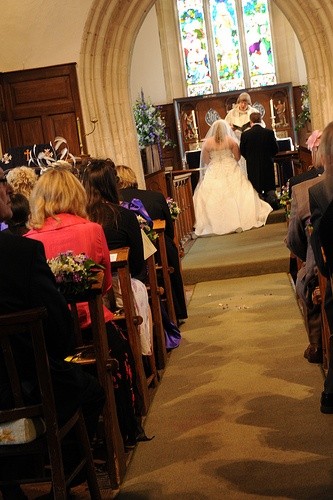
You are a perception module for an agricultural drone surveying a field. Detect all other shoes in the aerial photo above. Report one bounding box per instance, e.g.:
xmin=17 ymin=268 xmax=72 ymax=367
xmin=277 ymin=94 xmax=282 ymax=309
xmin=304 ymin=343 xmax=323 ymax=363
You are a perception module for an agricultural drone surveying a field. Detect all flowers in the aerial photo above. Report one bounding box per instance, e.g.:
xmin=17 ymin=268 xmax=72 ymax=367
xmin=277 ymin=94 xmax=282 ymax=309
xmin=136 ymin=214 xmax=160 ymax=242
xmin=276 ymin=180 xmax=293 ymax=219
xmin=294 ymin=84 xmax=311 ymax=131
xmin=46 ymin=249 xmax=106 ymax=296
xmin=305 ymin=130 xmax=323 ymax=151
xmin=166 ymin=196 xmax=181 ymax=220
xmin=305 ymin=217 xmax=314 ymax=237
xmin=134 ymin=91 xmax=178 ymax=150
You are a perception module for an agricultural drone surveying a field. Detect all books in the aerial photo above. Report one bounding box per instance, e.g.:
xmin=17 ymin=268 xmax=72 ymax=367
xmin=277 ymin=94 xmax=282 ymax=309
xmin=232 ymin=121 xmax=251 ymax=133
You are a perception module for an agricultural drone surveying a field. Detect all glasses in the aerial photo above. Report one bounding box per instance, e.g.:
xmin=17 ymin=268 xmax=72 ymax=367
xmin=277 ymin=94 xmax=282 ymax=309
xmin=0 ymin=176 xmax=7 ymax=184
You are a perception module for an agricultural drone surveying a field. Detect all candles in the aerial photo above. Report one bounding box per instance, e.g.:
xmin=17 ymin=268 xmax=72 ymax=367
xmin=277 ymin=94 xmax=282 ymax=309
xmin=191 ymin=110 xmax=196 ymax=128
xmin=269 ymin=99 xmax=274 ymax=117
xmin=76 ymin=116 xmax=83 ymax=146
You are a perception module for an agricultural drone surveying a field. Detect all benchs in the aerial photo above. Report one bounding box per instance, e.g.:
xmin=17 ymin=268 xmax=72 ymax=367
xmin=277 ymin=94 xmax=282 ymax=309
xmin=0 ymin=221 xmax=188 ymax=500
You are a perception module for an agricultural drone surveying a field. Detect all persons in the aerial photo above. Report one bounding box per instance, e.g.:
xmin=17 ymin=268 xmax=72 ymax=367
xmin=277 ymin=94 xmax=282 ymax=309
xmin=0 ymin=159 xmax=185 ymax=463
xmin=224 ymin=91 xmax=267 ymax=140
xmin=239 ymin=111 xmax=283 ymax=211
xmin=286 ymin=119 xmax=333 ymax=364
xmin=192 ymin=119 xmax=274 ymax=237
